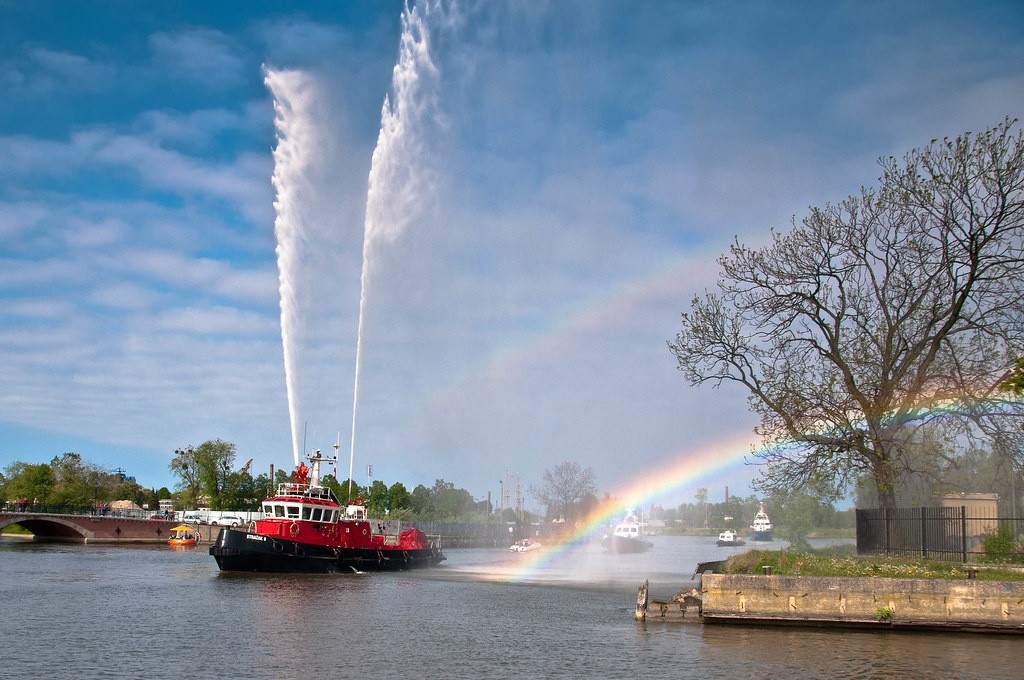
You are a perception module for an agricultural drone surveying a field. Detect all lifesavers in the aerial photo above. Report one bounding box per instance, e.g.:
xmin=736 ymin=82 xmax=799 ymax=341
xmin=294 ymin=547 xmax=306 ymax=559
xmin=382 ymin=557 xmax=392 ymax=569
xmin=290 ymin=523 xmax=299 ymax=535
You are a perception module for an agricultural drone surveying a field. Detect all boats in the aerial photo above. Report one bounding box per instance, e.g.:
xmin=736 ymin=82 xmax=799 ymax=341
xmin=749 ymin=500 xmax=774 ymax=542
xmin=167 ymin=522 xmax=202 ymax=548
xmin=602 ymin=506 xmax=657 ymax=555
xmin=716 ymin=525 xmax=747 ymax=547
xmin=508 ymin=538 xmax=543 ymax=554
xmin=209 ymin=421 xmax=448 ymax=576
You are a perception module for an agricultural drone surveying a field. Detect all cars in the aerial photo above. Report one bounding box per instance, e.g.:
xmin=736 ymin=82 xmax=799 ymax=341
xmin=179 ymin=514 xmax=207 ymax=525
xmin=207 ymin=514 xmax=245 ymax=529
xmin=147 ymin=514 xmax=172 ymax=522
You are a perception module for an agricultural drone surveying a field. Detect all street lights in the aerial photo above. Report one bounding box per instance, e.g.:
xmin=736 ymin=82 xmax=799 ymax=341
xmin=94 ymin=478 xmax=99 ymax=515
xmin=499 ymin=481 xmax=503 ymax=526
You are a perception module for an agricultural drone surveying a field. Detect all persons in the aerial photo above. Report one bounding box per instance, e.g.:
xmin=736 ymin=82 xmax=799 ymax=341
xmin=607 ymin=525 xmax=613 ymax=535
xmin=34 ymin=498 xmax=39 ymax=512
xmin=293 ymin=462 xmax=309 ymax=491
xmin=377 ymin=522 xmax=386 ymax=535
xmin=96 ymin=501 xmax=109 ymax=516
xmin=515 ymin=540 xmax=529 ymax=546
xmin=165 ymin=508 xmax=175 ymax=521
xmin=169 ymin=534 xmax=192 ymax=539
xmin=356 ymin=496 xmax=363 ymax=506
xmin=18 ymin=498 xmax=28 ymax=513
